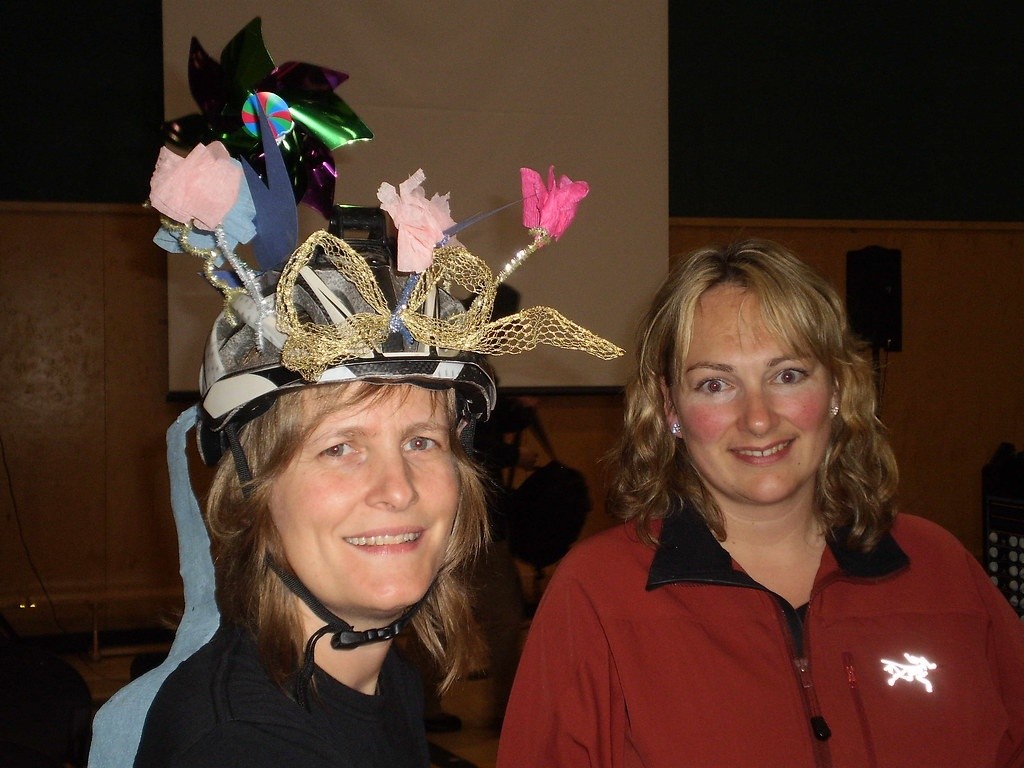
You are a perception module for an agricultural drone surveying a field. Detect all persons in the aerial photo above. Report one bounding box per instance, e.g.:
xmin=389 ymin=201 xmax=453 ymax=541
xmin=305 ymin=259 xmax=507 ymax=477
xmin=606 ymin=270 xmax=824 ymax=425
xmin=69 ymin=239 xmax=492 ymax=768
xmin=492 ymin=239 xmax=1024 ymax=768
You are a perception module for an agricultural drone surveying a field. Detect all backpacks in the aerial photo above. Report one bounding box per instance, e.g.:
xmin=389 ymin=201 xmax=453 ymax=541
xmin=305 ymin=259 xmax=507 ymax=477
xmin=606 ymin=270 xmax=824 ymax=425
xmin=501 ymin=407 xmax=592 ymax=570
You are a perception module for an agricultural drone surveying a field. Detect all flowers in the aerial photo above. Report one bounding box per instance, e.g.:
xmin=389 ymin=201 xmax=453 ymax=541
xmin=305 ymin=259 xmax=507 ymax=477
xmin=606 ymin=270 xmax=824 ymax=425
xmin=149 ymin=140 xmax=277 ymax=355
xmin=376 ymin=167 xmax=466 ymax=274
xmin=153 ymin=157 xmax=265 ymax=303
xmin=455 ymin=163 xmax=590 ymax=322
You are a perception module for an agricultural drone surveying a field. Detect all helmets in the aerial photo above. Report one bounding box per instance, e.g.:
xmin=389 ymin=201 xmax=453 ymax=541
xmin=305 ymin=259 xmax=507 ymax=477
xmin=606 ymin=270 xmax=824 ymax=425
xmin=194 ymin=264 xmax=500 ymax=469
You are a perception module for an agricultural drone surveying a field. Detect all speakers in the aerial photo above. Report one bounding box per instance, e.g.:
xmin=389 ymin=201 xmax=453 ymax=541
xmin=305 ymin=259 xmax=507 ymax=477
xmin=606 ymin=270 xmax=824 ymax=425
xmin=843 ymin=245 xmax=904 ymax=352
